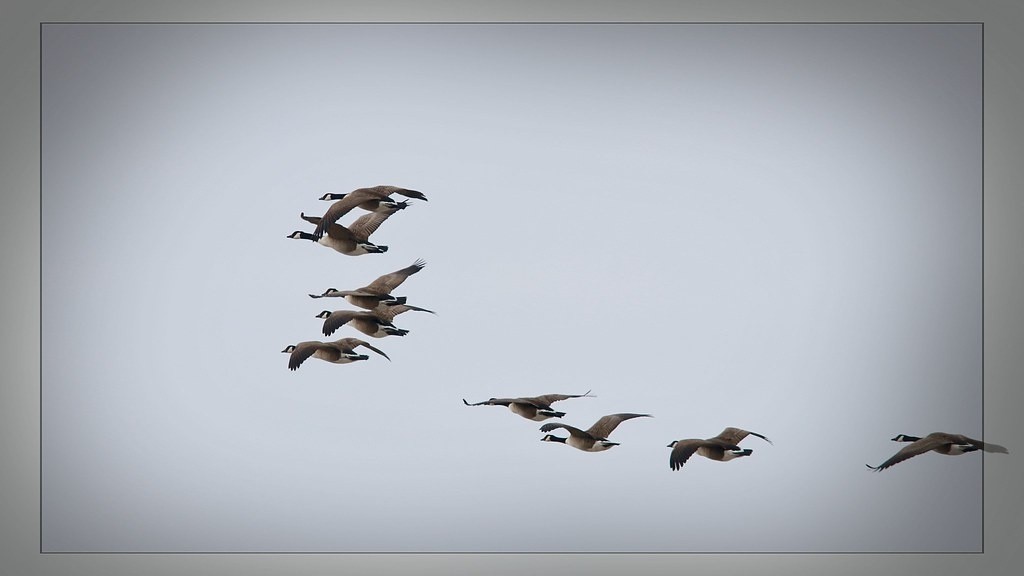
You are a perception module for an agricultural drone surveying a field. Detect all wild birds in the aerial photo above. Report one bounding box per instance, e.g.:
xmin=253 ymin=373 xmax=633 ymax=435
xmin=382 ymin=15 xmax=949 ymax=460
xmin=667 ymin=427 xmax=774 ymax=471
xmin=462 ymin=389 xmax=597 ymax=422
xmin=866 ymin=433 xmax=1009 ymax=472
xmin=287 ymin=186 xmax=428 ymax=256
xmin=539 ymin=413 xmax=655 ymax=453
xmin=281 ymin=258 xmax=437 ymax=372
